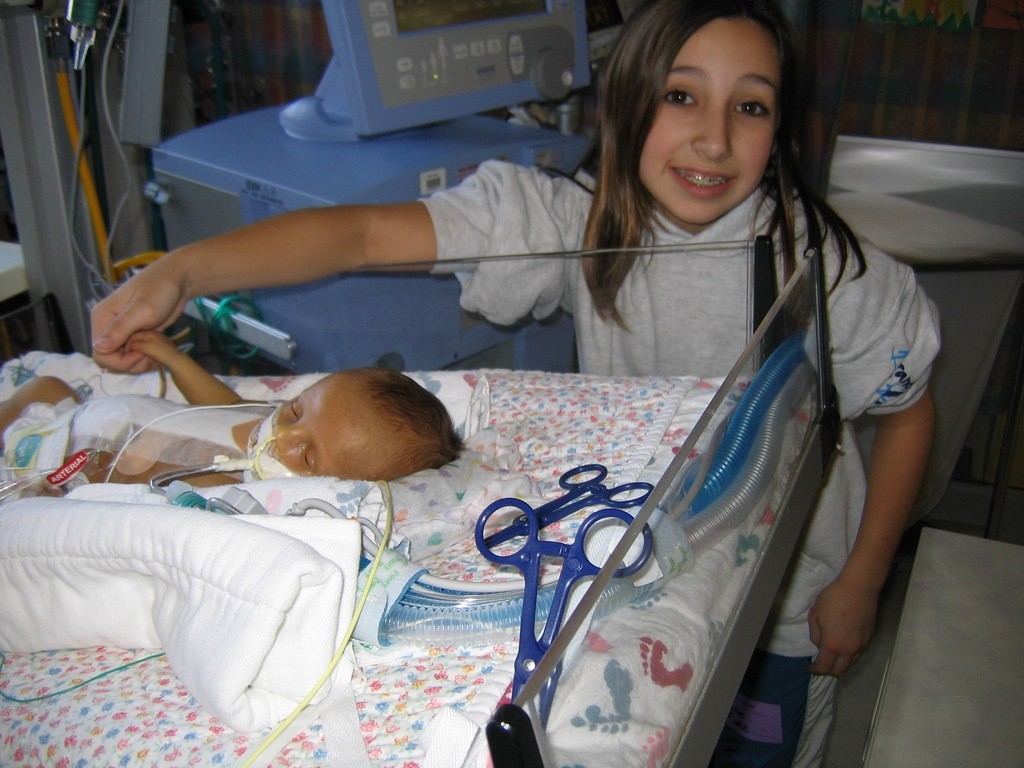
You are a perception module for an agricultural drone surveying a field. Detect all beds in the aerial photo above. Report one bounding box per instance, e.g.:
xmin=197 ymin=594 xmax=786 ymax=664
xmin=0 ymin=236 xmax=841 ymax=768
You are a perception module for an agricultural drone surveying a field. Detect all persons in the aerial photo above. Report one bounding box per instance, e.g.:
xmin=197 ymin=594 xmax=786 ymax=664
xmin=0 ymin=327 xmax=466 ymax=502
xmin=88 ymin=0 xmax=943 ymax=768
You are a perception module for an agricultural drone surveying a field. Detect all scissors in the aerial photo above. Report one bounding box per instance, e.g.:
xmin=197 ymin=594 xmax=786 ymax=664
xmin=472 ymin=496 xmax=655 ymax=731
xmin=478 ymin=463 xmax=658 ymax=550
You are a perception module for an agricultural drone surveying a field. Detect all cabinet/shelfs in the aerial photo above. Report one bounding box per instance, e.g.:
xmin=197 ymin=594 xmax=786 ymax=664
xmin=148 ymin=95 xmax=593 ymax=377
